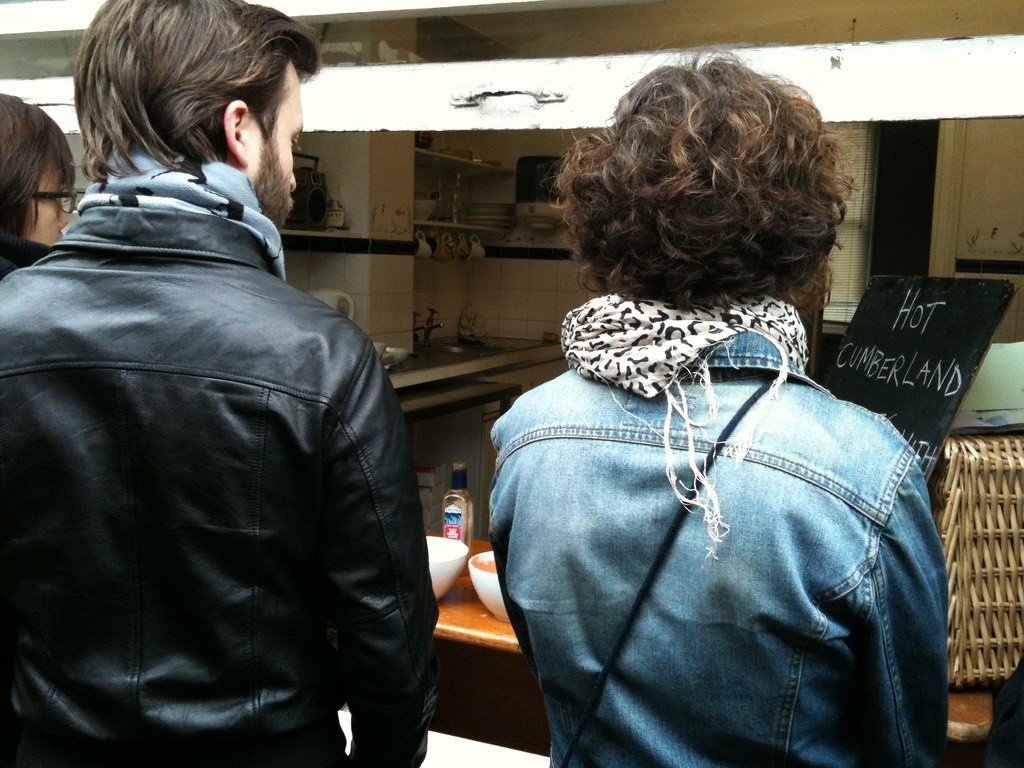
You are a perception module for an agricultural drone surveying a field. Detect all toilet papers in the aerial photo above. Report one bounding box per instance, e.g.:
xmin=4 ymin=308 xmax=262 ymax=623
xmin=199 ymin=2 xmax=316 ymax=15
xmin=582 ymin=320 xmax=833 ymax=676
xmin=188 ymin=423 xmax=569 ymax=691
xmin=304 ymin=285 xmax=361 ymax=327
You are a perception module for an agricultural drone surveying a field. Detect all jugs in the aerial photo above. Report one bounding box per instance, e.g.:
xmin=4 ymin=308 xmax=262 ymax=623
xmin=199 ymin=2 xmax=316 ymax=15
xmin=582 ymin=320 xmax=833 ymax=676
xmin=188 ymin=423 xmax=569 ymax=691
xmin=306 ymin=288 xmax=355 ymax=322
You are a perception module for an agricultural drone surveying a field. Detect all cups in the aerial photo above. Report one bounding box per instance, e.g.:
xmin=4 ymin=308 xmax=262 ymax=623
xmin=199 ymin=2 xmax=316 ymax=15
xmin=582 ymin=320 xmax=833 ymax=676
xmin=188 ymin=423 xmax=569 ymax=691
xmin=416 ymin=131 xmax=472 ymax=151
xmin=414 ymin=230 xmax=487 ymax=266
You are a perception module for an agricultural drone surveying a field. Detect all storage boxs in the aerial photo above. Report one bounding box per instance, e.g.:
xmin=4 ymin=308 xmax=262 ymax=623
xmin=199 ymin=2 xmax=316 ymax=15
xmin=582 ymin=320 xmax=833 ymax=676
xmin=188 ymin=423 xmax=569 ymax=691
xmin=926 ymin=432 xmax=1024 ymax=689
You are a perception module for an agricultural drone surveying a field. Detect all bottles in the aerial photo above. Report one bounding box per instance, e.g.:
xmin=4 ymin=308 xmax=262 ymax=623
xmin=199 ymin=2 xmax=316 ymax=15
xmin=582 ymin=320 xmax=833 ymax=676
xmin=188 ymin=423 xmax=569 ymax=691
xmin=447 ymin=168 xmax=466 ymax=225
xmin=441 ymin=462 xmax=473 ymax=576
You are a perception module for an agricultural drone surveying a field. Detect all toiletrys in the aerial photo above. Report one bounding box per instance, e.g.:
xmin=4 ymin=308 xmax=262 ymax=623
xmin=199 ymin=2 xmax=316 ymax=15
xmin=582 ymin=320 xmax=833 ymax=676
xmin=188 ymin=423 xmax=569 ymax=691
xmin=439 ymin=459 xmax=477 ymax=580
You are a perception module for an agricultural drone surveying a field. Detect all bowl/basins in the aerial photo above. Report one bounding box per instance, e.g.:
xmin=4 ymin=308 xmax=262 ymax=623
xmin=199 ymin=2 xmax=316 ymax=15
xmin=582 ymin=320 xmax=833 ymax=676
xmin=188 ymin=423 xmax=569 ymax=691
xmin=414 ymin=197 xmax=436 ymax=222
xmin=467 ymin=549 xmax=511 ymax=622
xmin=425 ymin=535 xmax=470 ymax=601
xmin=440 ymin=148 xmax=473 ymax=160
xmin=372 ymin=341 xmax=409 ymax=372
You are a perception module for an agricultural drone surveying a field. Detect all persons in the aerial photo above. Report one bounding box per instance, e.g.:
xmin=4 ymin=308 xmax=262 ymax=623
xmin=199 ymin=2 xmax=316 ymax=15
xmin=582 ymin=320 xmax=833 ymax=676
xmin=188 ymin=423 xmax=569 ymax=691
xmin=0 ymin=90 xmax=76 ymax=288
xmin=483 ymin=45 xmax=955 ymax=766
xmin=1 ymin=0 xmax=439 ymax=766
xmin=977 ymin=654 xmax=1024 ymax=768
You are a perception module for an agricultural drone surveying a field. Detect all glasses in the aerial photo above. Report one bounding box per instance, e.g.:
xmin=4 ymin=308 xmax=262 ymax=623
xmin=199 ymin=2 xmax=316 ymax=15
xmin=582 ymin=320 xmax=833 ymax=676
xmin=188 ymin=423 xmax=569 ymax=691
xmin=33 ymin=187 xmax=77 ymax=214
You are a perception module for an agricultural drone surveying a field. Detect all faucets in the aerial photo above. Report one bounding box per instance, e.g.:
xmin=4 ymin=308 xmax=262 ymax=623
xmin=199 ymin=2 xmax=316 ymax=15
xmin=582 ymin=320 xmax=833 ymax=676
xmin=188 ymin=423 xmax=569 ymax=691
xmin=413 ymin=310 xmax=430 ymax=348
xmin=425 ymin=308 xmax=445 ymax=345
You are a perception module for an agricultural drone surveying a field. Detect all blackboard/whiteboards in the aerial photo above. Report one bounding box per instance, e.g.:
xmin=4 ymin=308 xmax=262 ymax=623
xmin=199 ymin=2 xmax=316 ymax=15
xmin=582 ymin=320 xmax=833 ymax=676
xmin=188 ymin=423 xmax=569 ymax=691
xmin=819 ymin=274 xmax=1020 ymax=485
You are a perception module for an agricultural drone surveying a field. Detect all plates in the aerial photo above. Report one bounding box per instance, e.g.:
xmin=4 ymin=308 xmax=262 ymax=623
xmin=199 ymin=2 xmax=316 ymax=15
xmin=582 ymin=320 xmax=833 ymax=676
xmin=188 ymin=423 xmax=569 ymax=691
xmin=522 ymin=213 xmax=560 ymax=229
xmin=461 ymin=198 xmax=516 ymax=234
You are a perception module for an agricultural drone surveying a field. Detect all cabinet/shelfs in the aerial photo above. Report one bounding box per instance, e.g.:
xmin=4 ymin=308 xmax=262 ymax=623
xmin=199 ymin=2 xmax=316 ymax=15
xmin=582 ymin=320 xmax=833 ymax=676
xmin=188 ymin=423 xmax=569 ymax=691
xmin=412 ymin=17 xmax=529 ymax=244
xmin=927 ymin=119 xmax=1024 ymax=342
